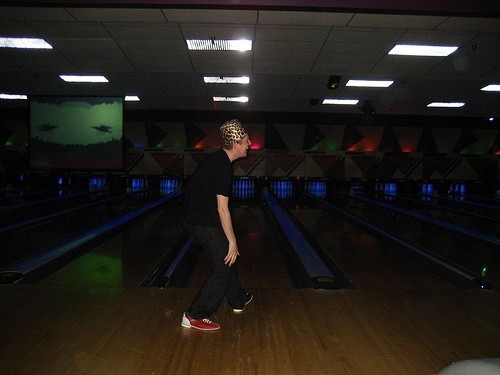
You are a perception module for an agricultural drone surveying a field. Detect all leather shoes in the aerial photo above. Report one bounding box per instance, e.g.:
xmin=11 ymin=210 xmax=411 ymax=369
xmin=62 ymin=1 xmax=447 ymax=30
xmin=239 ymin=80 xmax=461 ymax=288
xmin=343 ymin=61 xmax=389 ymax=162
xmin=181 ymin=311 xmax=220 ymax=330
xmin=233 ymin=293 xmax=253 ymax=312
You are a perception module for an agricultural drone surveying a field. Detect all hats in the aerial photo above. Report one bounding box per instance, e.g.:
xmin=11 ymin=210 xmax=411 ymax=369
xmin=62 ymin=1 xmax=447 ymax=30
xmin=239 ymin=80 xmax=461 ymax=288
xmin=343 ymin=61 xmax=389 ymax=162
xmin=220 ymin=119 xmax=248 ymax=146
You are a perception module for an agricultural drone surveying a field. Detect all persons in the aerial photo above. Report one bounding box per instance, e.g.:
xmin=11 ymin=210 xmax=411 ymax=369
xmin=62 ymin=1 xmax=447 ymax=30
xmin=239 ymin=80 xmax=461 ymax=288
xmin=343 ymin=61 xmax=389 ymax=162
xmin=181 ymin=118 xmax=253 ymax=330
